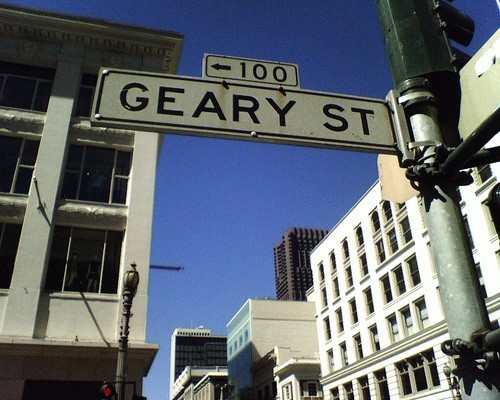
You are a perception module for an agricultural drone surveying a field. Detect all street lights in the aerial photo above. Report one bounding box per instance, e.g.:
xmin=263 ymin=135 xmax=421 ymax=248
xmin=444 ymin=362 xmax=462 ymax=400
xmin=115 ymin=263 xmax=138 ymax=400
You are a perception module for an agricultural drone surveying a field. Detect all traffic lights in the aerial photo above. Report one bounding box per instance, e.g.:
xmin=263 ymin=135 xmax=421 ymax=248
xmin=100 ymin=384 xmax=116 ymax=400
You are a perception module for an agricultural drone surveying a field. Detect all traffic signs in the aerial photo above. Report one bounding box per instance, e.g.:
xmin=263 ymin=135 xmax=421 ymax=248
xmin=89 ymin=53 xmax=400 ymax=155
